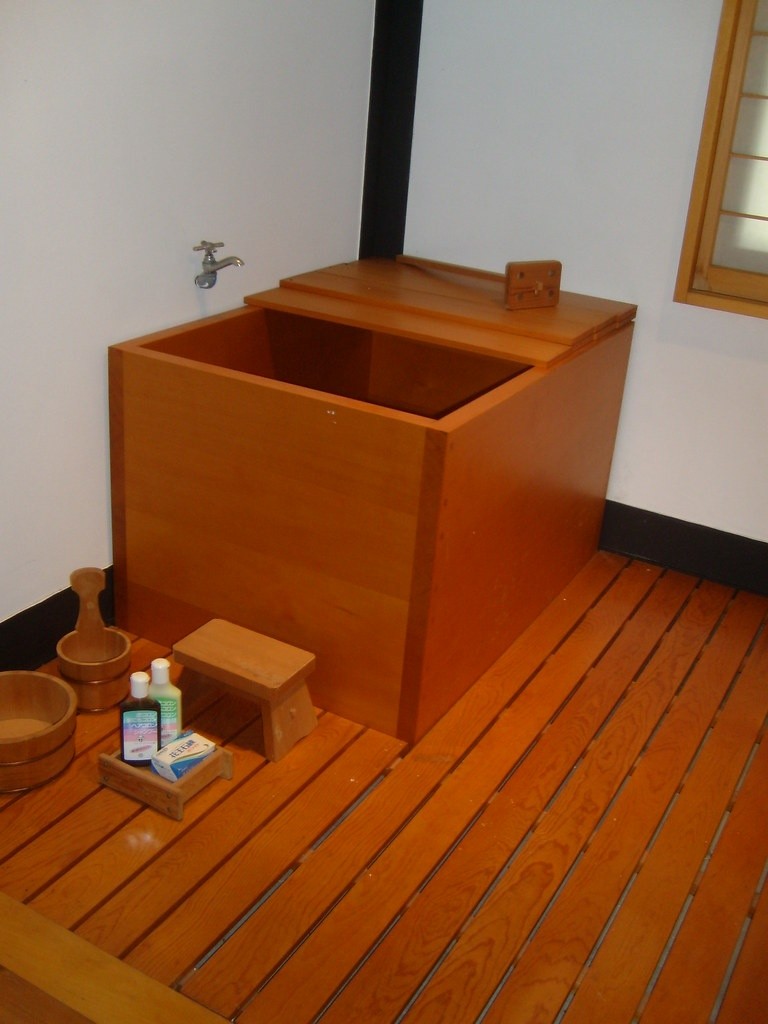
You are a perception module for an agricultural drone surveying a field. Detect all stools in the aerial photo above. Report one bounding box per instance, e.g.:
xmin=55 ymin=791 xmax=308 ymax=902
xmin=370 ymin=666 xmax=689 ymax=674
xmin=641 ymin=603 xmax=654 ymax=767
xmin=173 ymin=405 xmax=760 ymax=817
xmin=173 ymin=621 xmax=319 ymax=761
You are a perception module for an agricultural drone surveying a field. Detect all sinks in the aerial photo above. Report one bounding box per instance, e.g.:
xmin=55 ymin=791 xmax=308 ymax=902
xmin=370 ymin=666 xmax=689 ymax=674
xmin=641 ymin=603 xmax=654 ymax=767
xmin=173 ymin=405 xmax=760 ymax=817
xmin=107 ymin=297 xmax=542 ymax=435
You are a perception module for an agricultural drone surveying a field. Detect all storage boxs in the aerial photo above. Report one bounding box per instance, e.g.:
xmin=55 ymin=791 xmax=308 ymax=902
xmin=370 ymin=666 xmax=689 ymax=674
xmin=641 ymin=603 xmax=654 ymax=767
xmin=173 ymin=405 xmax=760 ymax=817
xmin=151 ymin=731 xmax=215 ymax=781
xmin=97 ymin=727 xmax=234 ymax=820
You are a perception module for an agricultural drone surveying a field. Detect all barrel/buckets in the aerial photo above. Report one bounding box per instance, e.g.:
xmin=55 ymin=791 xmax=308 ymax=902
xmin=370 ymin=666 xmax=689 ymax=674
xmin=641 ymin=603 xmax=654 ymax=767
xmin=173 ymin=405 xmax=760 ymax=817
xmin=0 ymin=669 xmax=78 ymax=796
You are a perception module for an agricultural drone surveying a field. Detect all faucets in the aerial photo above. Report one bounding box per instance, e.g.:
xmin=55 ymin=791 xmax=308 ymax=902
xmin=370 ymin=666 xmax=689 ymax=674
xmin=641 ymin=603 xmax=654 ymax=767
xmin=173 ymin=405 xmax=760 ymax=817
xmin=192 ymin=240 xmax=246 ymax=289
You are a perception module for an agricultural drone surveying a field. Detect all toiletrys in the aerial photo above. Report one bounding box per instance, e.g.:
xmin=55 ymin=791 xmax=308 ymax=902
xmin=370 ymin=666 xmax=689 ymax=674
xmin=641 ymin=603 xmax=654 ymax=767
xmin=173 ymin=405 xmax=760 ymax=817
xmin=149 ymin=658 xmax=181 ymax=749
xmin=120 ymin=671 xmax=162 ymax=767
xmin=149 ymin=728 xmax=216 ymax=783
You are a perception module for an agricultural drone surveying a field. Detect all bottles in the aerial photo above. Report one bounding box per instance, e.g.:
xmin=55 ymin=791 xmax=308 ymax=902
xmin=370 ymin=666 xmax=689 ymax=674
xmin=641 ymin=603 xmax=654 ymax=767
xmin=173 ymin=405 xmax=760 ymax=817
xmin=148 ymin=658 xmax=184 ymax=752
xmin=120 ymin=672 xmax=159 ymax=768
